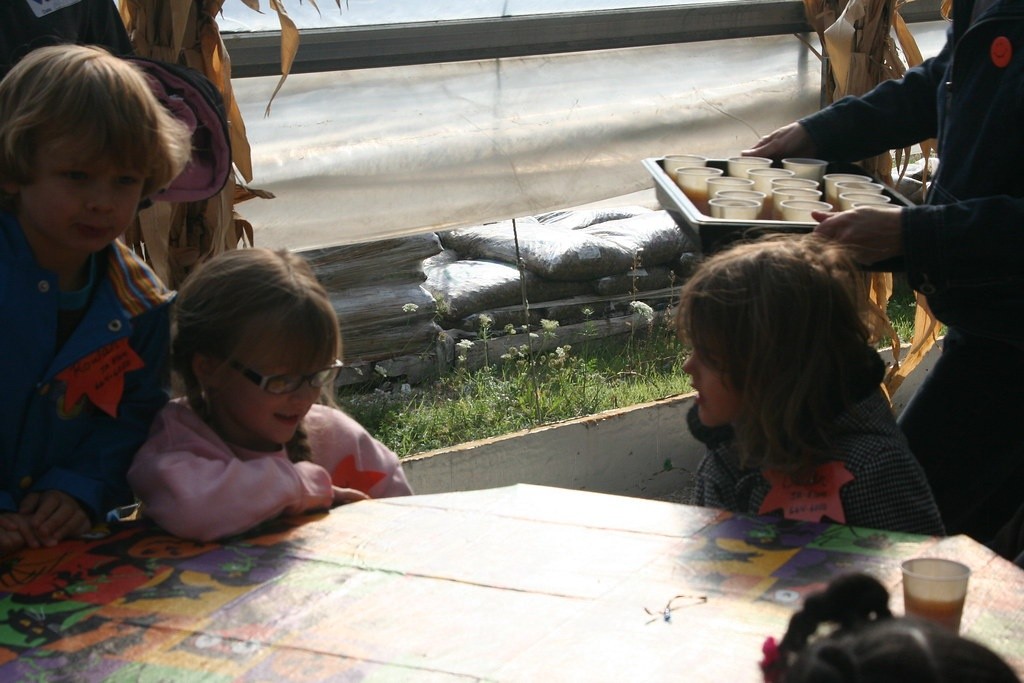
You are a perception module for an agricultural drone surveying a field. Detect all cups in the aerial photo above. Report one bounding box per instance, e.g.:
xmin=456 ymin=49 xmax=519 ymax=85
xmin=901 ymin=557 xmax=971 ymax=635
xmin=663 ymin=154 xmax=905 ymax=222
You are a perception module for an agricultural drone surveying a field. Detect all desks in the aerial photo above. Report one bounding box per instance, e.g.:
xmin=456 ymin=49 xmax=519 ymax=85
xmin=1 ymin=483 xmax=1023 ymax=683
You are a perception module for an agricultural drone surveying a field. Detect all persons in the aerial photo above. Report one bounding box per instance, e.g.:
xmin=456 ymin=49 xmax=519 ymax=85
xmin=122 ymin=245 xmax=416 ymax=545
xmin=741 ymin=0 xmax=1024 ymax=568
xmin=0 ymin=42 xmax=193 ymax=557
xmin=674 ymin=233 xmax=949 ymax=538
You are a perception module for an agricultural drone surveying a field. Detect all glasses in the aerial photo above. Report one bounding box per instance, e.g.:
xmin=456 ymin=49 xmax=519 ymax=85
xmin=223 ymin=356 xmax=344 ymax=394
